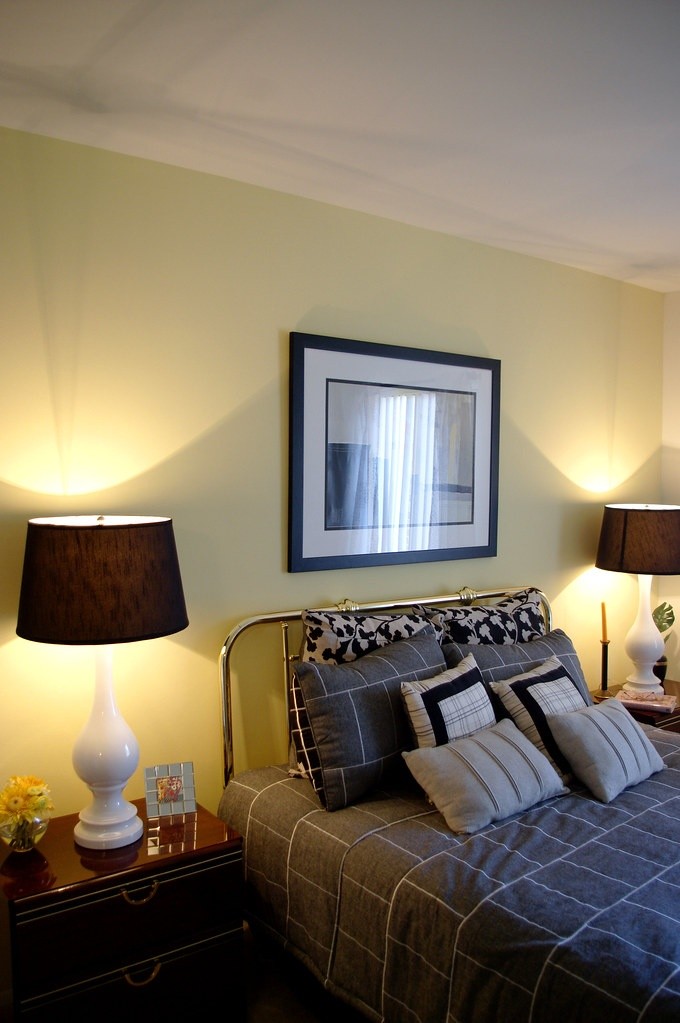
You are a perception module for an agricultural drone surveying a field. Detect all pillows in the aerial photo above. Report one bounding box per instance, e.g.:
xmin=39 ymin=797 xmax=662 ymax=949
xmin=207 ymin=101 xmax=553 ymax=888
xmin=413 ymin=587 xmax=546 ymax=647
xmin=288 ymin=609 xmax=447 ymax=779
xmin=401 ymin=717 xmax=571 ymax=834
xmin=441 ymin=629 xmax=594 ymax=706
xmin=546 ymin=697 xmax=670 ymax=804
xmin=489 ymin=654 xmax=588 ymax=785
xmin=288 ymin=625 xmax=447 ymax=814
xmin=400 ymin=652 xmax=496 ymax=805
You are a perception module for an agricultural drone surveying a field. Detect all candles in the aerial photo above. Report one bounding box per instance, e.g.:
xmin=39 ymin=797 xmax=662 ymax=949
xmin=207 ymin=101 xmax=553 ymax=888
xmin=601 ymin=600 xmax=607 ymax=641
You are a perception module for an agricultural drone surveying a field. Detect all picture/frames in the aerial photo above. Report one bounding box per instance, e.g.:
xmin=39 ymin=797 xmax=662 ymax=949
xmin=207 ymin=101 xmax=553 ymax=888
xmin=287 ymin=331 xmax=501 ymax=573
xmin=144 ymin=761 xmax=197 ymax=818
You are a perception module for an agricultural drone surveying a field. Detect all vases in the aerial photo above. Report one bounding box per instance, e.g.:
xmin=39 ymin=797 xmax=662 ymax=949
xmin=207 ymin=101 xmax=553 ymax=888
xmin=0 ymin=808 xmax=49 ymax=854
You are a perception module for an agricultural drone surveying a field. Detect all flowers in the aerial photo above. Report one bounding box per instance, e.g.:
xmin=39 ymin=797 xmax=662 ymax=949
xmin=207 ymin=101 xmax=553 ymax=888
xmin=0 ymin=771 xmax=54 ymax=846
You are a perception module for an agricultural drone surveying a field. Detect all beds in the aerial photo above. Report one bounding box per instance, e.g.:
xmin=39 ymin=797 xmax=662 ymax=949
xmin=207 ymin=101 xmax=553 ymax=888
xmin=218 ymin=588 xmax=679 ymax=1023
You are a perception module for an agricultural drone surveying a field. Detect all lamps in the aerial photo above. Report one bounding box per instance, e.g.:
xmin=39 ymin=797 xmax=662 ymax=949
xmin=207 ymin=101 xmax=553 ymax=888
xmin=16 ymin=516 xmax=189 ymax=853
xmin=595 ymin=504 xmax=680 ymax=693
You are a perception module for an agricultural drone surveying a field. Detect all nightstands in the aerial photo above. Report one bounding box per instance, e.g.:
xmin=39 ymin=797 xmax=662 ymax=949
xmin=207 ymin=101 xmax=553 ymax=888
xmin=0 ymin=797 xmax=250 ymax=1023
xmin=590 ymin=679 xmax=680 ymax=733
